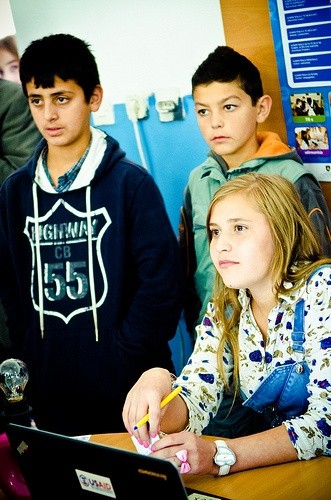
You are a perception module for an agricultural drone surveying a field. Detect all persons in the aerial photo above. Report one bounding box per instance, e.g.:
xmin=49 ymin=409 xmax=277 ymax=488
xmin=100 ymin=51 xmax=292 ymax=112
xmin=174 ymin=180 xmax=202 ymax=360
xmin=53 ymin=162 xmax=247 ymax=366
xmin=300 ymin=127 xmax=327 ymax=148
xmin=0 ymin=32 xmax=184 ymax=436
xmin=122 ymin=173 xmax=331 ymax=500
xmin=178 ymin=46 xmax=331 ymax=348
xmin=293 ymin=94 xmax=323 ymax=116
xmin=1 ymin=37 xmax=22 ymax=85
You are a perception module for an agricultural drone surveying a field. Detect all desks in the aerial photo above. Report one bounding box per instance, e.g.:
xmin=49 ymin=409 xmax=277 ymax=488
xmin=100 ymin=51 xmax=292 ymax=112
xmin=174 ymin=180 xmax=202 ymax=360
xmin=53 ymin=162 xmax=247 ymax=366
xmin=72 ymin=433 xmax=331 ymax=500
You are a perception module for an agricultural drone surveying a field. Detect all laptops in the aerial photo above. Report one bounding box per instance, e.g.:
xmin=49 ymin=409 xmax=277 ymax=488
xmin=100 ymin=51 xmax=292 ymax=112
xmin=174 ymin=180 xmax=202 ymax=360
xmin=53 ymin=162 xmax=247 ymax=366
xmin=6 ymin=424 xmax=232 ymax=500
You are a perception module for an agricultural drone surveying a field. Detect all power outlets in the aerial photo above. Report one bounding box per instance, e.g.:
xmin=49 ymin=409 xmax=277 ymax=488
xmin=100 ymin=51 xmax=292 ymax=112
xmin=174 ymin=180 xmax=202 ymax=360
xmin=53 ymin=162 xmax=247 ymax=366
xmin=156 ymin=90 xmax=182 ymax=122
xmin=127 ymin=96 xmax=148 ymax=119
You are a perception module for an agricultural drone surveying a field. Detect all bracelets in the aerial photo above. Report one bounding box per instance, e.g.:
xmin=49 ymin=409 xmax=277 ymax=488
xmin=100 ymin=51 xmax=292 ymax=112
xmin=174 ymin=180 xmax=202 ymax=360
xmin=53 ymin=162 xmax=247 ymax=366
xmin=214 ymin=439 xmax=236 ymax=477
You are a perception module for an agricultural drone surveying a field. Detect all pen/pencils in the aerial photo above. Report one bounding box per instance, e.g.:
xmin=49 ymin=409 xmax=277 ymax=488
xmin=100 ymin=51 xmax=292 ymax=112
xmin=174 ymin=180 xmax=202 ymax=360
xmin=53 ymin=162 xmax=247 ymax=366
xmin=132 ymin=386 xmax=182 ymax=430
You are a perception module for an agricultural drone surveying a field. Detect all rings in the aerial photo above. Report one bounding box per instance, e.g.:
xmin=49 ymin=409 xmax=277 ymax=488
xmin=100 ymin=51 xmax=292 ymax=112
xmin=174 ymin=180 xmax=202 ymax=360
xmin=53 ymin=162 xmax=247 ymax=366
xmin=176 ymin=450 xmax=191 ymax=473
